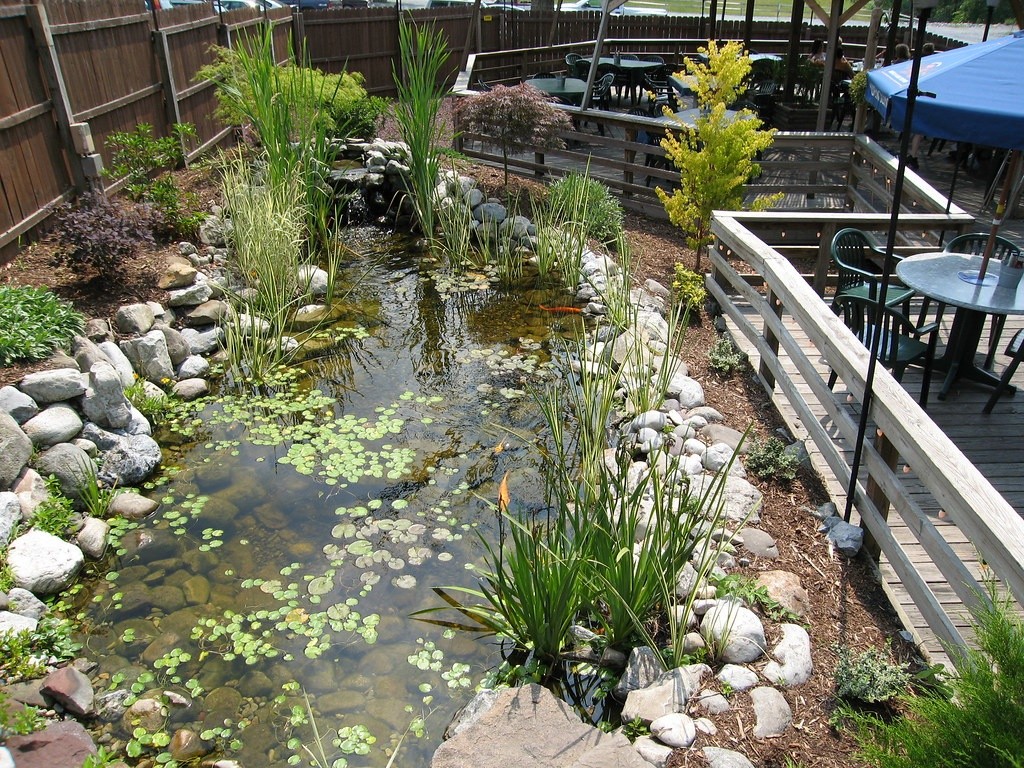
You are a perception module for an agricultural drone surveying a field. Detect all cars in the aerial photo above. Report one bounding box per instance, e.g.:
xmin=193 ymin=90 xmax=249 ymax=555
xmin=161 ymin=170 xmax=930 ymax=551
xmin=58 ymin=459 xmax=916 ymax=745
xmin=145 ymin=0 xmax=671 ymax=17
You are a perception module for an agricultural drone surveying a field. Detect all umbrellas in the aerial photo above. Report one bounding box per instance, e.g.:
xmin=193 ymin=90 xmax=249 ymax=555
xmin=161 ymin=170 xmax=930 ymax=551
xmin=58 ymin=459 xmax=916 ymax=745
xmin=864 ymin=28 xmax=1024 ymax=281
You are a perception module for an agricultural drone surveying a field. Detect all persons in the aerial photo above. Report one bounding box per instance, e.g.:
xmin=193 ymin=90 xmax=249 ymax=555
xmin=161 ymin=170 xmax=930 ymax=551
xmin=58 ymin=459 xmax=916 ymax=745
xmin=809 ymin=35 xmax=935 ymax=170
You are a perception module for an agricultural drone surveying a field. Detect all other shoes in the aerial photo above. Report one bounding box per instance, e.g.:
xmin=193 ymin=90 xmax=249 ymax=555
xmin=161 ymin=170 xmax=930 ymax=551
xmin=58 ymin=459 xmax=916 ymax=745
xmin=907 ymin=155 xmax=920 ymax=170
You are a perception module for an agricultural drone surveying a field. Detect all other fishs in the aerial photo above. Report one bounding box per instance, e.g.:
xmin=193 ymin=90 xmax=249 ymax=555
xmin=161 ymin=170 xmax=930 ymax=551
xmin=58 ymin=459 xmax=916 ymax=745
xmin=539 ymin=304 xmax=580 ymax=312
xmin=497 ymin=470 xmax=511 ymax=511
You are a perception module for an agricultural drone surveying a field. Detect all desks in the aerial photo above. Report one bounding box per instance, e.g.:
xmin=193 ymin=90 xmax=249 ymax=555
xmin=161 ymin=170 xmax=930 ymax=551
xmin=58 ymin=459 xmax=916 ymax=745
xmin=524 ymin=78 xmax=586 ymax=105
xmin=897 ymin=251 xmax=1024 ymax=401
xmin=579 ymin=57 xmax=663 ymax=105
xmin=652 ymin=108 xmax=756 ymax=135
xmin=668 ymin=74 xmax=739 ymax=105
xmin=699 ymin=54 xmax=783 ymax=90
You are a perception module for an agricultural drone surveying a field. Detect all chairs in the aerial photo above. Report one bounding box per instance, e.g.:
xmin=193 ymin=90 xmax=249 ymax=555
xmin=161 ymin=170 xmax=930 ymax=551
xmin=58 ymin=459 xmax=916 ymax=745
xmin=475 ymin=51 xmax=880 ymax=193
xmin=828 ymin=227 xmax=1024 ymax=413
